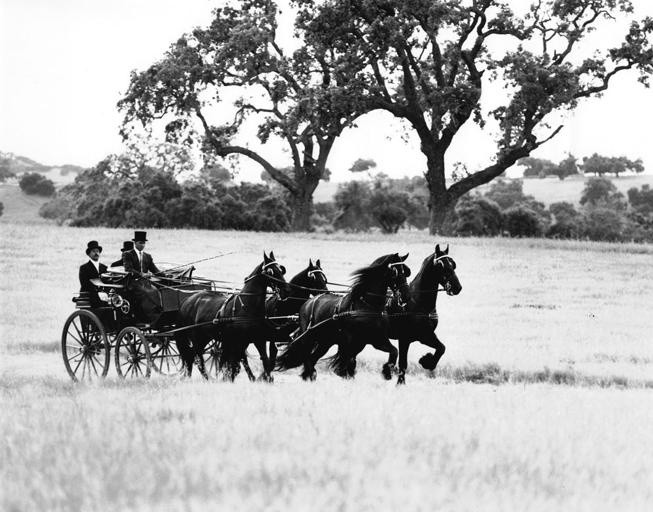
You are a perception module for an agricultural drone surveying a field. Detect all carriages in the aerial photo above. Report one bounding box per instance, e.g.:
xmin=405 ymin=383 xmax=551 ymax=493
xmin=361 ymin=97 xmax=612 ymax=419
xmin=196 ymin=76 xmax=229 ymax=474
xmin=58 ymin=249 xmax=330 ymax=385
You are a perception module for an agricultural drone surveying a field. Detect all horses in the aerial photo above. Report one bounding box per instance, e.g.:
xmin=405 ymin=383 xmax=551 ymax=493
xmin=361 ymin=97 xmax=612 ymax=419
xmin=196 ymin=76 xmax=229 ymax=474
xmin=170 ymin=249 xmax=286 ymax=383
xmin=274 ymin=250 xmax=412 ymax=383
xmin=211 ymin=255 xmax=331 ymax=380
xmin=325 ymin=243 xmax=463 ymax=379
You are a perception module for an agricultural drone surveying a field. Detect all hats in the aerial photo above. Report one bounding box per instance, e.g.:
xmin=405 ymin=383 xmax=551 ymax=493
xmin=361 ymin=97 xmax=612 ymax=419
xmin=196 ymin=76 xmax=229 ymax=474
xmin=132 ymin=231 xmax=149 ymax=242
xmin=85 ymin=240 xmax=103 ymax=256
xmin=120 ymin=241 xmax=134 ymax=251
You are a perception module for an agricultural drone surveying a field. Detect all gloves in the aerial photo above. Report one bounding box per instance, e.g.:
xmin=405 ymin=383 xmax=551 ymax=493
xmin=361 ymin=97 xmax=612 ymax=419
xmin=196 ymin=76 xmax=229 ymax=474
xmin=99 ymin=292 xmax=110 ymax=302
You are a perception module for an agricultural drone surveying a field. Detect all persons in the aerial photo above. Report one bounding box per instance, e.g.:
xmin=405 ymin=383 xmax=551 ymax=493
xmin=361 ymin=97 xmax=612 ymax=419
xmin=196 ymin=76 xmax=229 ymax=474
xmin=121 ymin=229 xmax=172 ymax=282
xmin=110 ymin=241 xmax=133 ymax=267
xmin=77 ymin=239 xmax=108 ymax=296
